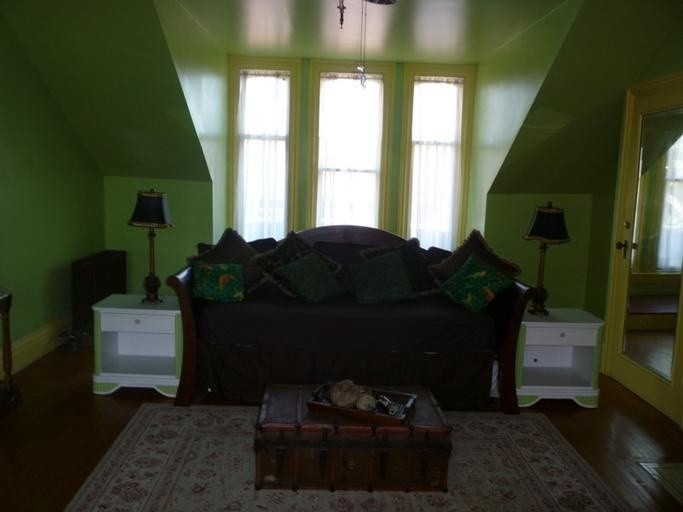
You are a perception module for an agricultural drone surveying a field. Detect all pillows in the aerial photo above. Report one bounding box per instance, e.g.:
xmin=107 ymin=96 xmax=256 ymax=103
xmin=209 ymin=227 xmax=266 ymax=289
xmin=439 ymin=253 xmax=515 ymax=311
xmin=187 ymin=249 xmax=222 ymax=263
xmin=362 ymin=238 xmax=440 ymax=299
xmin=346 ymin=249 xmax=411 ymax=305
xmin=270 ymin=248 xmax=344 ymax=306
xmin=190 ymin=261 xmax=243 ymax=305
xmin=251 ymin=228 xmax=343 ymax=299
xmin=427 ymin=228 xmax=522 ymax=286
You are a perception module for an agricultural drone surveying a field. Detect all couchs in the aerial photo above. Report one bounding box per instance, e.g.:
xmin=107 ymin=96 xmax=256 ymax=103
xmin=164 ymin=225 xmax=534 ymax=415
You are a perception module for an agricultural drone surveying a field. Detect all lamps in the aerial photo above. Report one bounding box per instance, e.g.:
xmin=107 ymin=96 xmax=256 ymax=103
xmin=522 ymin=199 xmax=570 ymax=318
xmin=126 ymin=188 xmax=174 ymax=304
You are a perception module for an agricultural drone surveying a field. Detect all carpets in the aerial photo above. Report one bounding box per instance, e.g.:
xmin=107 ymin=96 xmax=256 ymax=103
xmin=64 ymin=400 xmax=638 ymax=510
xmin=635 ymin=458 xmax=682 ymax=507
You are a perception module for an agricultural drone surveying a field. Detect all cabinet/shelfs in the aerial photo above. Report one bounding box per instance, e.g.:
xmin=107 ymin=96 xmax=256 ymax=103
xmin=91 ymin=294 xmax=183 ymax=399
xmin=516 ymin=308 xmax=607 ymax=409
xmin=70 ymin=250 xmax=126 ymax=334
